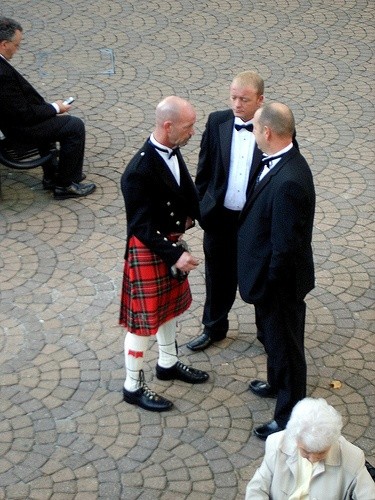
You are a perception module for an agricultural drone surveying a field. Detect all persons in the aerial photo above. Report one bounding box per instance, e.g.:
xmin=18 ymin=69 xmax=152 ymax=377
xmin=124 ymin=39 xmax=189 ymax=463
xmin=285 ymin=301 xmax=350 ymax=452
xmin=244 ymin=397 xmax=375 ymax=500
xmin=186 ymin=71 xmax=300 ymax=351
xmin=119 ymin=95 xmax=210 ymax=412
xmin=0 ymin=16 xmax=96 ymax=201
xmin=236 ymin=102 xmax=315 ymax=441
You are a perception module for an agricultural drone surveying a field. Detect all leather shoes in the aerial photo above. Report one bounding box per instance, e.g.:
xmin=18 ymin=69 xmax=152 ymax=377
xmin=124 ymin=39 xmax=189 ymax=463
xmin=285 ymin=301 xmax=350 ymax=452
xmin=253 ymin=419 xmax=285 ymax=441
xmin=249 ymin=379 xmax=277 ymax=398
xmin=42 ymin=172 xmax=86 ymax=190
xmin=185 ymin=333 xmax=226 ymax=351
xmin=155 ymin=361 xmax=209 ymax=384
xmin=53 ymin=182 xmax=96 ymax=200
xmin=123 ymin=385 xmax=173 ymax=413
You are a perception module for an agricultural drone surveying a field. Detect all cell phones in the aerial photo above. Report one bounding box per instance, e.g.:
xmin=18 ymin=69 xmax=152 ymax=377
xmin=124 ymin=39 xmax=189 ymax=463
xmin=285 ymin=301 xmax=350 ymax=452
xmin=63 ymin=97 xmax=75 ymax=105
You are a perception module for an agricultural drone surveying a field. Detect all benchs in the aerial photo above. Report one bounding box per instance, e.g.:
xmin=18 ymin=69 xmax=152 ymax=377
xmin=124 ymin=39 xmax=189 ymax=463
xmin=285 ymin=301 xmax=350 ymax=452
xmin=0 ymin=140 xmax=59 ymax=169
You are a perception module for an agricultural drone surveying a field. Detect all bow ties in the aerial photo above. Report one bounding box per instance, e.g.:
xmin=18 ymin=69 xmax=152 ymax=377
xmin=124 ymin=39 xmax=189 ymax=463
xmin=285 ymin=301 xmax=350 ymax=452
xmin=262 ymin=152 xmax=282 ymax=168
xmin=234 ymin=123 xmax=253 ymax=132
xmin=149 ymin=140 xmax=180 ymax=159
